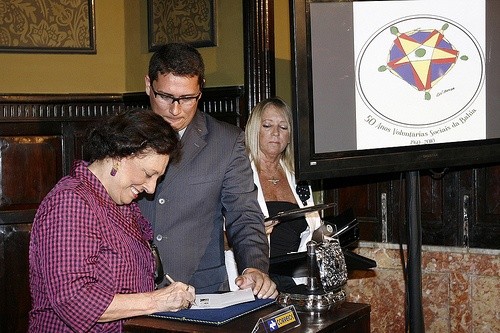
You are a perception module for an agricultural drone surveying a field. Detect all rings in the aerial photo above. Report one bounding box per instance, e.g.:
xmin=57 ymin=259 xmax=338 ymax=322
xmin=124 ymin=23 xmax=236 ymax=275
xmin=185 ymin=284 xmax=190 ymax=290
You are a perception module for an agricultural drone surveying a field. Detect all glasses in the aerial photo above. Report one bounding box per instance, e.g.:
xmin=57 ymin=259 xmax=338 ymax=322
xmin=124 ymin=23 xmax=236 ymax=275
xmin=149 ymin=81 xmax=200 ymax=105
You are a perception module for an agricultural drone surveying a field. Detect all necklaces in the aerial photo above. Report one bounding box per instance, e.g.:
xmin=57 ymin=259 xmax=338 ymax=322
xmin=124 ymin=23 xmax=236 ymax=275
xmin=261 ymin=170 xmax=281 ymax=184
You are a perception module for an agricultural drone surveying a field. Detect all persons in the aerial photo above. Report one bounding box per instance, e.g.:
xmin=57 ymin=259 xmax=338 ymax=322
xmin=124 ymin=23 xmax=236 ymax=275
xmin=224 ymin=96 xmax=322 ymax=291
xmin=29 ymin=107 xmax=195 ymax=333
xmin=136 ymin=42 xmax=279 ymax=299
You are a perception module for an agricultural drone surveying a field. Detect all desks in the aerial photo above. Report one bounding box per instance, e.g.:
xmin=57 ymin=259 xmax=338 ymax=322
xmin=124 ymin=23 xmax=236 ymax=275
xmin=123 ymin=292 xmax=371 ymax=333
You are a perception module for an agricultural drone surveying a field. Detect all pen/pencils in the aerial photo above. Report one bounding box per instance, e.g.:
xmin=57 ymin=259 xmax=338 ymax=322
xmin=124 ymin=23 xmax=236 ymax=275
xmin=164 ymin=273 xmax=198 ymax=306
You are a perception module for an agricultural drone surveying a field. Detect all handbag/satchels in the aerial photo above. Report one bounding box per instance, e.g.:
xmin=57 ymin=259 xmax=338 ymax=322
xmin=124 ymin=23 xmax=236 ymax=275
xmin=306 ymin=225 xmax=348 ymax=289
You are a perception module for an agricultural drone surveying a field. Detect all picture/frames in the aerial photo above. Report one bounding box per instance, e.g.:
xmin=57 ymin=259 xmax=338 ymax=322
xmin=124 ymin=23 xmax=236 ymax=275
xmin=147 ymin=0 xmax=217 ymax=52
xmin=0 ymin=0 xmax=97 ymax=55
